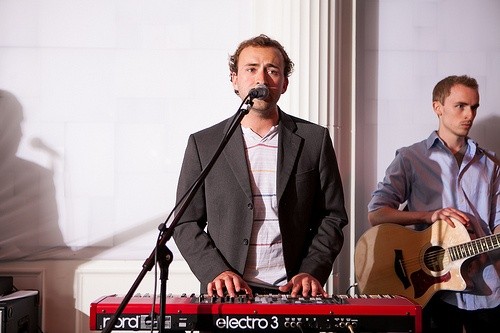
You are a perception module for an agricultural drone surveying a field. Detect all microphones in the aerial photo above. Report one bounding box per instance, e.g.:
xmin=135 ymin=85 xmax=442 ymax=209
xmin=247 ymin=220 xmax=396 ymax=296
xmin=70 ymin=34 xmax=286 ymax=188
xmin=248 ymin=84 xmax=269 ymax=100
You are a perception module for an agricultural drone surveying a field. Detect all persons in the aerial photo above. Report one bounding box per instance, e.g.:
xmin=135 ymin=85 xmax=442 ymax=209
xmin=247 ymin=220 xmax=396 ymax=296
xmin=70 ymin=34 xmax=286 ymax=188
xmin=366 ymin=74 xmax=500 ymax=333
xmin=171 ymin=33 xmax=349 ymax=298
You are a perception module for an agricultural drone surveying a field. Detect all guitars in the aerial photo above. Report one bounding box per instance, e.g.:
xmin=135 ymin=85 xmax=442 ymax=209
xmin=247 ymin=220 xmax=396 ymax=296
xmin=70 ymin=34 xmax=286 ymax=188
xmin=353 ymin=213 xmax=500 ymax=311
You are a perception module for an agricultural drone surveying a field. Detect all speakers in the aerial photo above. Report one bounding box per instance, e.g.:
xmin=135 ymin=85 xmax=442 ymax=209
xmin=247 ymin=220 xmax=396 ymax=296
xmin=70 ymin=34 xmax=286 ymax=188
xmin=0 ymin=290 xmax=41 ymax=333
